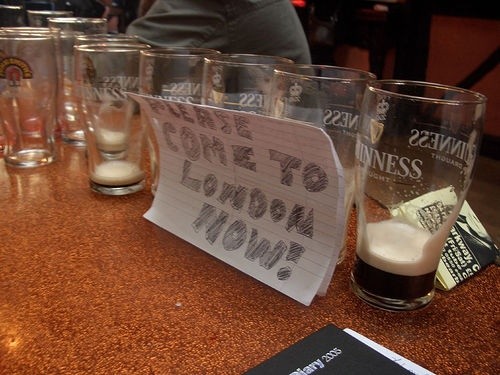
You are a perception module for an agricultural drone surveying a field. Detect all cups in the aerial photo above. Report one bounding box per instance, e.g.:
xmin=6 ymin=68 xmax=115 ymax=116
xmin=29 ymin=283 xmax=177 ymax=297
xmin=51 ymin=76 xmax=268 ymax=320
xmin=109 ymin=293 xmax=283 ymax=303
xmin=270 ymin=65 xmax=376 ymax=265
xmin=139 ymin=48 xmax=220 ymax=198
xmin=202 ymin=53 xmax=292 ymax=118
xmin=351 ymin=79 xmax=487 ymax=312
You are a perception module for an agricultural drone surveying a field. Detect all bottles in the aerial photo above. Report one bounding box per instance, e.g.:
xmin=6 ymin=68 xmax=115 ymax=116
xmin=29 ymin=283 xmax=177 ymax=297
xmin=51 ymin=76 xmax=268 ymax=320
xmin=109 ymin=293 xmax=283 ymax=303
xmin=0 ymin=4 xmax=151 ymax=196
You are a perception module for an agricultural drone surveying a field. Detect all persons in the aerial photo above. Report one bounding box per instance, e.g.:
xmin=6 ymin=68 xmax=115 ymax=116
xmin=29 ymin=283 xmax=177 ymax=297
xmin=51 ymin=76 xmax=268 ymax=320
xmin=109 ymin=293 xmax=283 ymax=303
xmin=23 ymin=0 xmax=434 ymax=132
